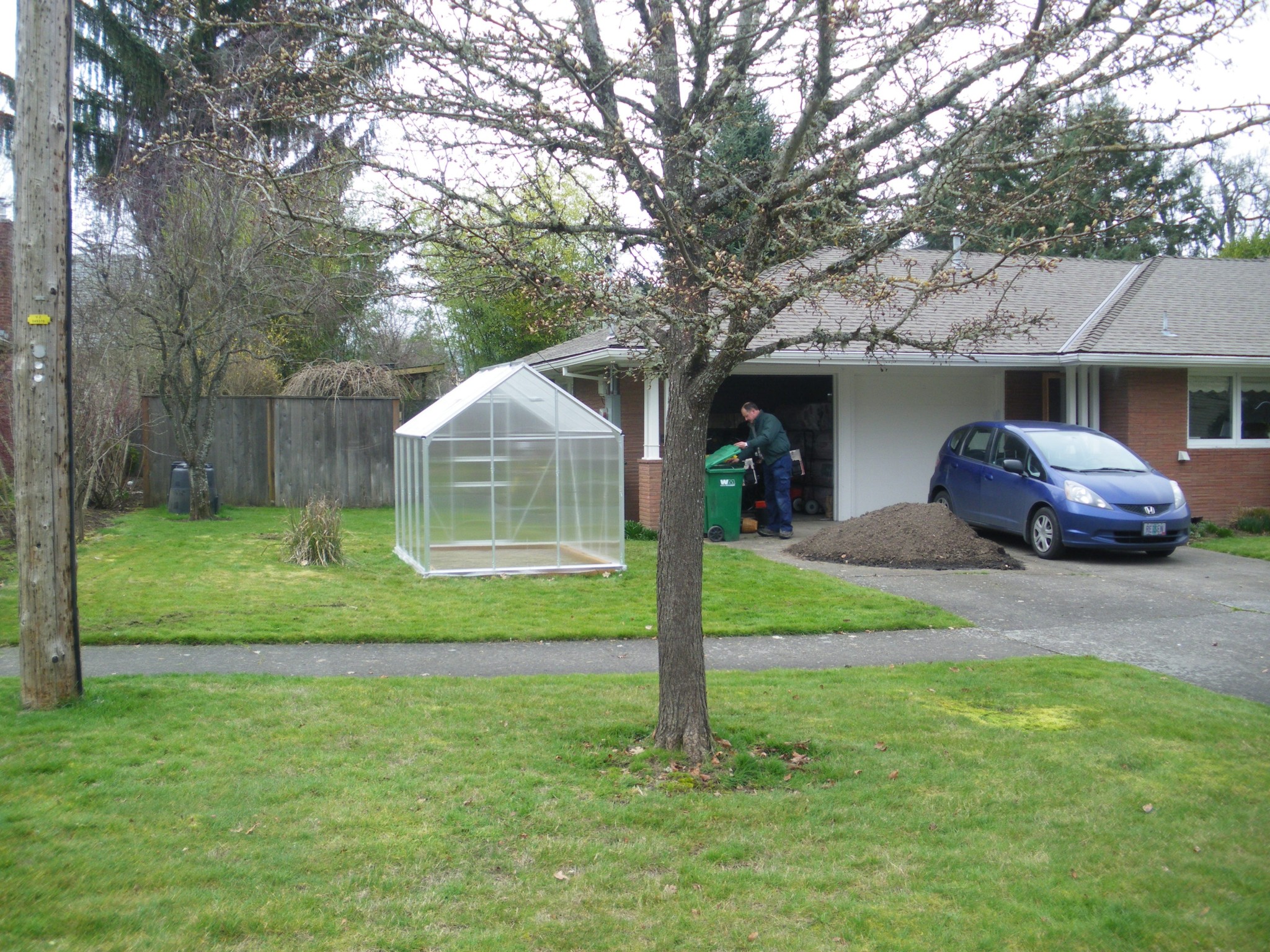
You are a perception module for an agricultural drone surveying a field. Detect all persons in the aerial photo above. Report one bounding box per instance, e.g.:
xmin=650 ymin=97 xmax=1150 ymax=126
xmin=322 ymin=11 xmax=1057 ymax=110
xmin=726 ymin=402 xmax=793 ymax=538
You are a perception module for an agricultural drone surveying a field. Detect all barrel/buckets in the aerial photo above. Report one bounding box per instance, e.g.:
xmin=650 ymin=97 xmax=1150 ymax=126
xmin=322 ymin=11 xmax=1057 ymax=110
xmin=168 ymin=462 xmax=219 ymax=514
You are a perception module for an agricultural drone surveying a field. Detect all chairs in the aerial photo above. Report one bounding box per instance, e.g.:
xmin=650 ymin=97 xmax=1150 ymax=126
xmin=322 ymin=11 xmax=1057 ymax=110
xmin=971 ymin=437 xmax=1023 ymax=465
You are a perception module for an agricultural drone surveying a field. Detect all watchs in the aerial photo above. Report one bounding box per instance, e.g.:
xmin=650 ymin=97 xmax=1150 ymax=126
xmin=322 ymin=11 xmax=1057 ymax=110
xmin=745 ymin=443 xmax=748 ymax=448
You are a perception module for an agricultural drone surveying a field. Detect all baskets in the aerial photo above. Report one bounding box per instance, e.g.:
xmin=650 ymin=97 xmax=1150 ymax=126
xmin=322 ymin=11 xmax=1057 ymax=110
xmin=741 ymin=517 xmax=758 ymax=533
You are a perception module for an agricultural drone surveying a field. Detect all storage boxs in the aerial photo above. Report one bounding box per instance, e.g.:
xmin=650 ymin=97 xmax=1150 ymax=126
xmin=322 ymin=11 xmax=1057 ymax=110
xmin=755 ymin=501 xmax=768 ymax=526
xmin=791 ymin=486 xmax=804 ymax=501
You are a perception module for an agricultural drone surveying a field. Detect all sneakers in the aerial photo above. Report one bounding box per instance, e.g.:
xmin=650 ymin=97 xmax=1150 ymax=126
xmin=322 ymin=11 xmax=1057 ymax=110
xmin=757 ymin=528 xmax=779 ymax=536
xmin=779 ymin=530 xmax=794 ymax=538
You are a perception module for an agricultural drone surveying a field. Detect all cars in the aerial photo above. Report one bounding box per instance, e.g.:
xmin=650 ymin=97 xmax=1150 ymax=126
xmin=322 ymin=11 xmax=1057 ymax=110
xmin=926 ymin=418 xmax=1191 ymax=560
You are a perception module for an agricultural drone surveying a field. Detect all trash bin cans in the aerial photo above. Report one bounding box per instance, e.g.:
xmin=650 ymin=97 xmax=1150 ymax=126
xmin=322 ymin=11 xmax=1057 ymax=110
xmin=703 ymin=444 xmax=746 ymax=542
